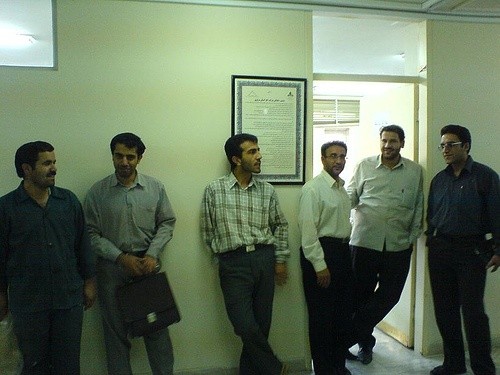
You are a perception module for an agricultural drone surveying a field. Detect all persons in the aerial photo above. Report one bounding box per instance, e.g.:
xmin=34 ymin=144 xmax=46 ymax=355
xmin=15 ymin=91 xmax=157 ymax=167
xmin=346 ymin=125 xmax=425 ymax=364
xmin=0 ymin=140 xmax=96 ymax=375
xmin=297 ymin=141 xmax=358 ymax=375
xmin=426 ymin=125 xmax=500 ymax=375
xmin=83 ymin=132 xmax=176 ymax=375
xmin=199 ymin=134 xmax=292 ymax=375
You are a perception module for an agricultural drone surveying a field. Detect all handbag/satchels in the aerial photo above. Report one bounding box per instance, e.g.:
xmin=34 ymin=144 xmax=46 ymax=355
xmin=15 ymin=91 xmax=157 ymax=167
xmin=116 ymin=272 xmax=181 ymax=338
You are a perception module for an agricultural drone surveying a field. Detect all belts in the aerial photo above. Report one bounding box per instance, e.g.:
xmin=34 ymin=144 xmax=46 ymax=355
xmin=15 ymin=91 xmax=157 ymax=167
xmin=224 ymin=244 xmax=269 ymax=256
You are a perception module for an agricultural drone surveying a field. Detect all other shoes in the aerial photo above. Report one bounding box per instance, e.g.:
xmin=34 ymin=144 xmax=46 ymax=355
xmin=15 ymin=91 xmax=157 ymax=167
xmin=358 ymin=346 xmax=372 ymax=364
xmin=430 ymin=366 xmax=461 ymax=375
xmin=346 ymin=351 xmax=357 ymax=360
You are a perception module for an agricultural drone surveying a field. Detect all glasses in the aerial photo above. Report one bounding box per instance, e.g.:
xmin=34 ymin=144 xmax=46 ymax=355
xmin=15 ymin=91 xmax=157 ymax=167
xmin=437 ymin=142 xmax=463 ymax=151
xmin=326 ymin=154 xmax=348 ymax=161
